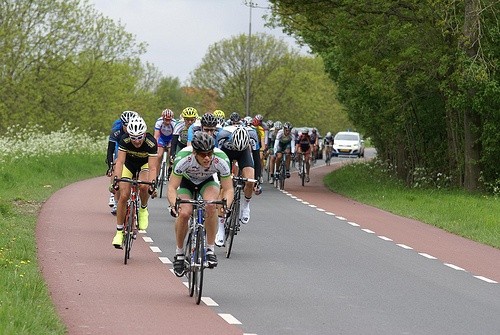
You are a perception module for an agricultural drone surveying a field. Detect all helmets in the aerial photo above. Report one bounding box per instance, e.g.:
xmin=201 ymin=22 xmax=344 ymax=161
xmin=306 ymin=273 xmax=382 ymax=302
xmin=120 ymin=111 xmax=138 ymax=123
xmin=162 ymin=106 xmax=332 ymax=153
xmin=127 ymin=116 xmax=147 ymax=135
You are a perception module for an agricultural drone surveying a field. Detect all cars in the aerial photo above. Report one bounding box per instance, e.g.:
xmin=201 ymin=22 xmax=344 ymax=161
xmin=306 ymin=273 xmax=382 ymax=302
xmin=332 ymin=132 xmax=365 ymax=159
xmin=291 ymin=127 xmax=323 ymax=160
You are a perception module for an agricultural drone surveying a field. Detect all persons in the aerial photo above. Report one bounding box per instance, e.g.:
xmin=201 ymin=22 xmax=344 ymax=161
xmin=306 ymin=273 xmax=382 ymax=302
xmin=153 ymin=108 xmax=176 ymax=176
xmin=112 ymin=116 xmax=159 ymax=245
xmin=321 ymin=132 xmax=334 ymax=162
xmin=215 ymin=125 xmax=262 ymax=246
xmin=170 ymin=107 xmax=319 ymax=183
xmin=107 ymin=110 xmax=139 ymax=215
xmin=168 ymin=131 xmax=234 ymax=277
xmin=188 ymin=112 xmax=232 ymax=150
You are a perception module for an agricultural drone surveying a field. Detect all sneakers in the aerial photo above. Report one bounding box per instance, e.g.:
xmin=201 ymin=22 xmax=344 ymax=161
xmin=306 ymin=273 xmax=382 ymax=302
xmin=174 ymin=253 xmax=185 ymax=277
xmin=138 ymin=207 xmax=148 ymax=230
xmin=112 ymin=231 xmax=124 ymax=247
xmin=206 ymin=250 xmax=217 ymax=266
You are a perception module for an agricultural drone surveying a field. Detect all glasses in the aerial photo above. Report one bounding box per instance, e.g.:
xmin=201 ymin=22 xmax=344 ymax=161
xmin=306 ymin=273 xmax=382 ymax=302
xmin=184 ymin=118 xmax=196 ymax=122
xmin=203 ymin=128 xmax=216 ymax=131
xmin=129 ymin=134 xmax=144 ymax=140
xmin=198 ymin=150 xmax=213 ymax=157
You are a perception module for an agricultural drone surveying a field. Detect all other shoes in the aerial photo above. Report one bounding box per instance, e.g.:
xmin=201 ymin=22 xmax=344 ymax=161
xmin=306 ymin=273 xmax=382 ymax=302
xmin=215 ymin=229 xmax=225 ymax=247
xmin=269 ymin=176 xmax=274 ymax=183
xmin=109 ymin=195 xmax=115 ymax=206
xmin=112 ymin=207 xmax=117 ymax=215
xmin=286 ymin=173 xmax=290 ymax=178
xmin=306 ymin=175 xmax=309 ymax=182
xmin=241 ymin=205 xmax=250 ymax=224
xmin=260 ymin=176 xmax=263 ymax=183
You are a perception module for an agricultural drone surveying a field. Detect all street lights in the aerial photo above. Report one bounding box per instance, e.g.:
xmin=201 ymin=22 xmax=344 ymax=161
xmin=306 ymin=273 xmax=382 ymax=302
xmin=244 ymin=1 xmax=258 ymax=116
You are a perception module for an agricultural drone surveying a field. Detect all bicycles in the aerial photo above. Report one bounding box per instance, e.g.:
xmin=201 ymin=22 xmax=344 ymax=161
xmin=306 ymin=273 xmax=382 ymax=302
xmin=223 ymin=176 xmax=264 ymax=259
xmin=158 ymin=145 xmax=170 ymax=198
xmin=175 ymin=197 xmax=231 ymax=304
xmin=297 ymin=153 xmax=310 ymax=186
xmin=324 ymin=145 xmax=333 ymax=166
xmin=262 ymin=150 xmax=294 ymax=190
xmin=112 ymin=176 xmax=156 ymax=265
